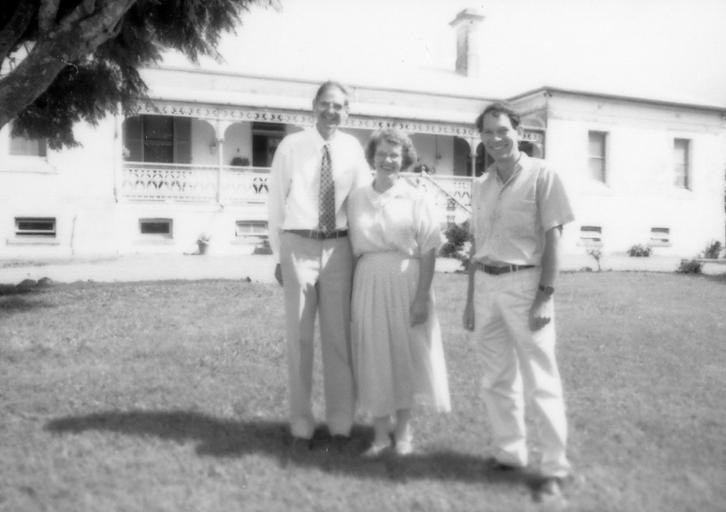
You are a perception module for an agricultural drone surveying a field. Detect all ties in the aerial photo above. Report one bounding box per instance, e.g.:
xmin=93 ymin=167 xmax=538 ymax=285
xmin=318 ymin=144 xmax=336 ymax=237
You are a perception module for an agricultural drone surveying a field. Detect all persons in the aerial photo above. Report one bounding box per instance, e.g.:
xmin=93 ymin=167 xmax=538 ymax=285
xmin=463 ymin=105 xmax=576 ymax=502
xmin=266 ymin=81 xmax=374 ymax=451
xmin=346 ymin=127 xmax=452 ymax=460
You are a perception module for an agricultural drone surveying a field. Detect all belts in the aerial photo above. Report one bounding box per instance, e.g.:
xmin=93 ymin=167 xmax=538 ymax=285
xmin=476 ymin=261 xmax=533 ymax=274
xmin=290 ymin=227 xmax=346 ymax=240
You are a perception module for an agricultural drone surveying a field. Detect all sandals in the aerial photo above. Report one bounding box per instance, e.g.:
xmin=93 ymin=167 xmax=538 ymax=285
xmin=394 ymin=427 xmax=412 ymax=456
xmin=359 ymin=441 xmax=391 ymax=460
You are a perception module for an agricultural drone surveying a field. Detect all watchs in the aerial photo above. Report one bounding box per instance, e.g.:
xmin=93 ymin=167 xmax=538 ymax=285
xmin=539 ymin=285 xmax=555 ymax=295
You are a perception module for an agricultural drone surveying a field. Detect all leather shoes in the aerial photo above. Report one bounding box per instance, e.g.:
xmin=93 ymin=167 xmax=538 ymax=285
xmin=330 ymin=435 xmax=348 ymax=455
xmin=483 ymin=458 xmax=511 ymax=471
xmin=533 ymin=476 xmax=563 ymax=502
xmin=291 ymin=439 xmax=313 ymax=456
